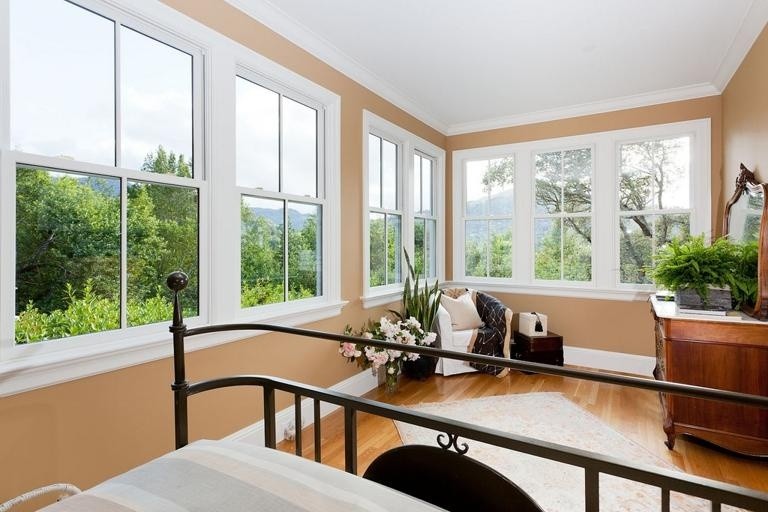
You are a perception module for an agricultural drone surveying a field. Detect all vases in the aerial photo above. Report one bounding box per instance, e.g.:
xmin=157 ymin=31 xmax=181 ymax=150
xmin=386 ymin=363 xmax=398 ymax=393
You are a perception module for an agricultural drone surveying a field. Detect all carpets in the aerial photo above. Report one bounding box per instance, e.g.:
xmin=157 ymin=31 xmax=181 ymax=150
xmin=394 ymin=391 xmax=747 ymax=511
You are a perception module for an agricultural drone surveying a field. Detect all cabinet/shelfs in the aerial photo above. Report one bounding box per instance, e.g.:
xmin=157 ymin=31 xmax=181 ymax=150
xmin=514 ymin=328 xmax=564 ymax=374
xmin=646 ymin=292 xmax=768 ymax=462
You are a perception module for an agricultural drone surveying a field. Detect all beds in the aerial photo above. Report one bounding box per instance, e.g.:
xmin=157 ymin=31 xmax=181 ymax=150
xmin=34 ymin=270 xmax=767 ymax=511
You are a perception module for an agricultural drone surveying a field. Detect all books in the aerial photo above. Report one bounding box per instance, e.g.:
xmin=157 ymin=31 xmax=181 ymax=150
xmin=677 ymin=304 xmax=742 ymax=323
xmin=655 ymin=290 xmax=675 ymax=301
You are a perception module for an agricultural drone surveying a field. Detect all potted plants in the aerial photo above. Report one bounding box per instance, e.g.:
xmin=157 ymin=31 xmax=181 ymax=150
xmin=387 ymin=246 xmax=443 ymax=381
xmin=637 ymin=232 xmax=748 ymax=312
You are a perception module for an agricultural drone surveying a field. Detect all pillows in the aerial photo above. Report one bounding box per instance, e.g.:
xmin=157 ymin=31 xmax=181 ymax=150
xmin=438 ymin=290 xmax=485 ymax=331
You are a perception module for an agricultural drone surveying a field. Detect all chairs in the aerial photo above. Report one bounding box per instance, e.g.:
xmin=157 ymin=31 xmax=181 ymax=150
xmin=429 ymin=286 xmax=514 ymax=376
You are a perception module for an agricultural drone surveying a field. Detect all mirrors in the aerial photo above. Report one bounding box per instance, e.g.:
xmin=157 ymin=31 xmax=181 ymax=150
xmin=722 ymin=162 xmax=768 ymax=314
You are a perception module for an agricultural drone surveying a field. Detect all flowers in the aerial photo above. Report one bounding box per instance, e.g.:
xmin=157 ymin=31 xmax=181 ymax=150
xmin=338 ymin=315 xmax=437 ymax=375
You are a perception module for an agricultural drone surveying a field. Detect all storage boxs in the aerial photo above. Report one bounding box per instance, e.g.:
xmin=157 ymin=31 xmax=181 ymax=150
xmin=519 ymin=312 xmax=548 ymax=337
